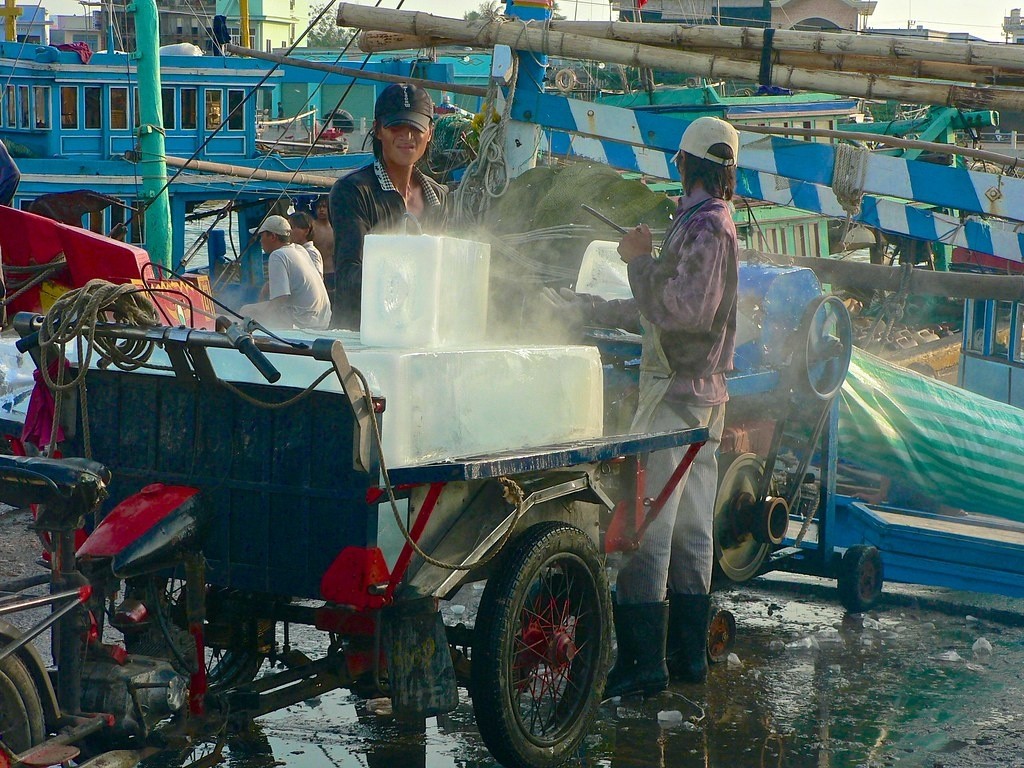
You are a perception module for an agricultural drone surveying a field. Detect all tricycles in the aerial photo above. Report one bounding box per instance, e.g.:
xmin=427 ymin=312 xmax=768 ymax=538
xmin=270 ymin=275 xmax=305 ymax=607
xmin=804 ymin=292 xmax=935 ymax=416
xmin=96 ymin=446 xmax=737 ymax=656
xmin=1 ymin=308 xmax=713 ymax=768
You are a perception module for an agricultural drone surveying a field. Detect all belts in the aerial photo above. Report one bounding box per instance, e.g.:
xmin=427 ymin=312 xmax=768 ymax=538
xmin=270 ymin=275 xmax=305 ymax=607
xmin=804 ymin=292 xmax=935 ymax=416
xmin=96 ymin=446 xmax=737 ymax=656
xmin=663 ymin=400 xmax=701 ymax=428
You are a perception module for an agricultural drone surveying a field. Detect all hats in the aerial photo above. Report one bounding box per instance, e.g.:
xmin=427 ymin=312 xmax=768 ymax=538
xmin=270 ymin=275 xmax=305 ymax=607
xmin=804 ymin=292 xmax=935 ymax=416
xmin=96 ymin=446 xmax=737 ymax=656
xmin=248 ymin=215 xmax=290 ymax=237
xmin=670 ymin=116 xmax=739 ymax=167
xmin=374 ymin=82 xmax=433 ymax=134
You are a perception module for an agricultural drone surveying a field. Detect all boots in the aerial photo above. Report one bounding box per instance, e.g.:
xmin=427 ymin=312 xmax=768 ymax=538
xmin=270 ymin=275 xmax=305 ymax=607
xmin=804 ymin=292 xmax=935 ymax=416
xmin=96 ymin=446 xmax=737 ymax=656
xmin=599 ymin=589 xmax=671 ymax=702
xmin=664 ymin=592 xmax=711 ymax=684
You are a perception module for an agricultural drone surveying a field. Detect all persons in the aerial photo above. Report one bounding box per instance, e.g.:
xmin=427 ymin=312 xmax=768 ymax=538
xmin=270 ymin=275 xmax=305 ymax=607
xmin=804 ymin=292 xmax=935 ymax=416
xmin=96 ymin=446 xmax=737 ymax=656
xmin=603 ymin=117 xmax=738 ymax=696
xmin=329 ymin=84 xmax=447 ymax=332
xmin=241 ymin=196 xmax=333 ymax=330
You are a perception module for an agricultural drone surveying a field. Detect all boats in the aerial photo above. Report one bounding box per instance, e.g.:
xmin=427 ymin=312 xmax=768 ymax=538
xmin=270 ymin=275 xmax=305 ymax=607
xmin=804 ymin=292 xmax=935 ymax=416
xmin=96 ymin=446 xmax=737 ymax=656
xmin=0 ymin=40 xmax=1024 ymax=388
